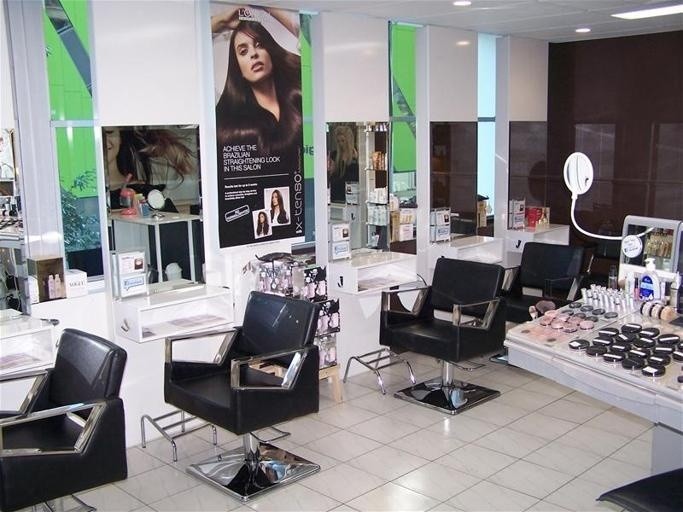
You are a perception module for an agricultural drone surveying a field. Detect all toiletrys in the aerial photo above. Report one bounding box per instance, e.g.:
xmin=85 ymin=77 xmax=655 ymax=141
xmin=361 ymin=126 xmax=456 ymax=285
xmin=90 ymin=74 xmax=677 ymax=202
xmin=578 ymin=282 xmax=634 ymax=313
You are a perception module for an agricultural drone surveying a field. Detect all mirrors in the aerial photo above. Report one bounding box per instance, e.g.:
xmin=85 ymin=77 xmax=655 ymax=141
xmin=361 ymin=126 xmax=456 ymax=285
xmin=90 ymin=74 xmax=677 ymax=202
xmin=0 ymin=132 xmax=30 ymax=324
xmin=102 ymin=124 xmax=205 ymax=295
xmin=322 ymin=121 xmax=390 ymax=255
xmin=574 ymin=125 xmax=616 ymax=211
xmin=426 ymin=120 xmax=478 ymax=240
xmin=507 ymin=122 xmax=547 ymax=223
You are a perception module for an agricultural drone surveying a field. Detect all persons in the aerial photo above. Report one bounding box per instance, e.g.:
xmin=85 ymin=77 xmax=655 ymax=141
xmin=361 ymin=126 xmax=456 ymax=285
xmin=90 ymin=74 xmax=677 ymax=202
xmin=269 ymin=189 xmax=288 ymax=226
xmin=255 ymin=211 xmax=270 ymax=235
xmin=328 ymin=125 xmax=358 ymax=205
xmin=101 ymin=126 xmax=197 ymax=214
xmin=210 ymin=4 xmax=303 ymax=174
xmin=536 ymin=207 xmax=543 ymax=221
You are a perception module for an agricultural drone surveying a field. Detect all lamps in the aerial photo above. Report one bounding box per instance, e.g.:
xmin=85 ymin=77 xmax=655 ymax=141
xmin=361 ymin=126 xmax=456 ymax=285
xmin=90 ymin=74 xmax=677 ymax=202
xmin=563 ymin=150 xmax=654 ymax=240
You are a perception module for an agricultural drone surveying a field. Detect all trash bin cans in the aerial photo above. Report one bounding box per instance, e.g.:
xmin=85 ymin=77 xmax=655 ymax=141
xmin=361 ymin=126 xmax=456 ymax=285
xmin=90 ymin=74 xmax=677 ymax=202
xmin=164 ymin=263 xmax=182 ymax=281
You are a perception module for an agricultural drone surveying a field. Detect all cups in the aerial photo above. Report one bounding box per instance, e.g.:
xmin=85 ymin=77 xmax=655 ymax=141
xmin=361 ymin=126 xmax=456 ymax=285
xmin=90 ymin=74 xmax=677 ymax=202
xmin=527 ymin=208 xmax=537 ymax=228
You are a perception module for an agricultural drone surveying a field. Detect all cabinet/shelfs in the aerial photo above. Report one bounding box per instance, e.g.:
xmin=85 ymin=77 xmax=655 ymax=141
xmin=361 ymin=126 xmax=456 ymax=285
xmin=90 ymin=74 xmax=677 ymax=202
xmin=262 ymin=253 xmax=343 ymax=406
xmin=328 ymin=249 xmax=418 ymax=296
xmin=420 ymin=235 xmax=501 ymax=270
xmin=503 ymin=225 xmax=569 ymax=253
xmin=502 ymin=214 xmax=683 ymax=438
xmin=117 ymin=285 xmax=236 ymax=343
xmin=1 ymin=317 xmax=56 ymax=377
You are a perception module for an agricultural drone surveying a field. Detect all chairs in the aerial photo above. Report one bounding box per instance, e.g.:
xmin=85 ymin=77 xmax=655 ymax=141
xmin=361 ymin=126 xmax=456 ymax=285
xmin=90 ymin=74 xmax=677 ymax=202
xmin=505 ymin=242 xmax=583 ymax=325
xmin=381 ymin=252 xmax=505 ymax=415
xmin=588 ymin=235 xmax=646 ymax=285
xmin=2 ymin=328 xmax=129 ymax=511
xmin=595 ymin=466 xmax=683 ymax=512
xmin=164 ymin=290 xmax=321 ymax=501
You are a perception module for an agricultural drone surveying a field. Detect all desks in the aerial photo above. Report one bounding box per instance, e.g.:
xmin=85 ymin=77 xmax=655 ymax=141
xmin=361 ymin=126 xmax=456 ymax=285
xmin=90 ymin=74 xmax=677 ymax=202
xmin=106 ymin=208 xmax=200 ymax=286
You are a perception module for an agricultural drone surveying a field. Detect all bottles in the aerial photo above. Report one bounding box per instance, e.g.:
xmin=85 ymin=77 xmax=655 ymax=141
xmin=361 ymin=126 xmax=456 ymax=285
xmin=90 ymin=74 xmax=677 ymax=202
xmin=48 ymin=274 xmax=61 ymax=300
